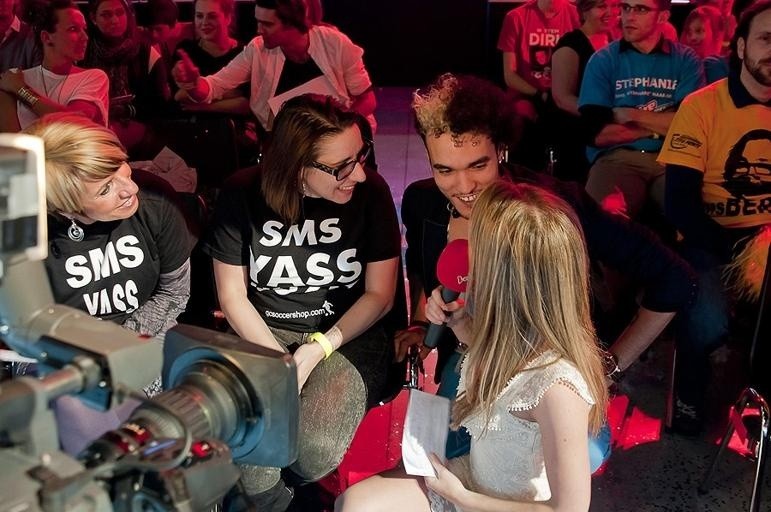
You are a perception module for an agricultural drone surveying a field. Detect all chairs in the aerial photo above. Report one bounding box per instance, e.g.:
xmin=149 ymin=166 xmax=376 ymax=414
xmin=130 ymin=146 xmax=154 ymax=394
xmin=703 ymin=240 xmax=771 ymax=510
xmin=374 ymin=339 xmax=419 ymax=407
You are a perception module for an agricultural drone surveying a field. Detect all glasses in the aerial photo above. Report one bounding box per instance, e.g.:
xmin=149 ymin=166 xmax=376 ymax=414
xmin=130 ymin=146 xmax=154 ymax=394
xmin=304 ymin=136 xmax=374 ymax=182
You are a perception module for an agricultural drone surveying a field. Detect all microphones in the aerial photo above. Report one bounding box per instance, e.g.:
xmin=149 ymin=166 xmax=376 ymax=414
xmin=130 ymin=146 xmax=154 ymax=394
xmin=423 ymin=239 xmax=469 ymax=349
